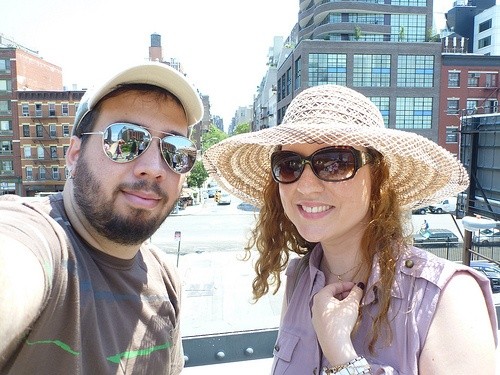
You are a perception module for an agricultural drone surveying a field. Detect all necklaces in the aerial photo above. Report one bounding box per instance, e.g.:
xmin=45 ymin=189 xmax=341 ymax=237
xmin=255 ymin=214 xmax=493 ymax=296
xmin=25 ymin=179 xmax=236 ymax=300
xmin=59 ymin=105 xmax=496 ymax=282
xmin=323 ymin=257 xmax=363 ymax=281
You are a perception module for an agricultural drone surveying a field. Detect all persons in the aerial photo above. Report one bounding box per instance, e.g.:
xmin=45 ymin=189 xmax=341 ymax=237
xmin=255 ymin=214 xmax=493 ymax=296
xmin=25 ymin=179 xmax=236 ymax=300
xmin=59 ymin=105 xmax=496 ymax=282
xmin=162 ymin=147 xmax=193 ymax=173
xmin=203 ymin=84 xmax=498 ymax=375
xmin=423 ymin=220 xmax=429 ymax=231
xmin=334 ymin=161 xmax=339 ymax=174
xmin=104 ymin=136 xmax=149 ymax=159
xmin=0 ymin=62 xmax=203 ymax=375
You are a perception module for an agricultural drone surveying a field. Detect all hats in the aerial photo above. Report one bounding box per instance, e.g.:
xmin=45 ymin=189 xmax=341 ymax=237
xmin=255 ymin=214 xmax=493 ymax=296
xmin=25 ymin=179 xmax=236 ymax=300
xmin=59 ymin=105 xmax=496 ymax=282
xmin=70 ymin=63 xmax=204 ymax=142
xmin=202 ymin=84 xmax=470 ymax=211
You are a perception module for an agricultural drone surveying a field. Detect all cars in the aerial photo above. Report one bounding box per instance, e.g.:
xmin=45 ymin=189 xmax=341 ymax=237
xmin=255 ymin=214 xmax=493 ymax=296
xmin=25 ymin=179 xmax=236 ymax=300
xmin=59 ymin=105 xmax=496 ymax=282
xmin=208 ymin=182 xmax=231 ymax=205
xmin=453 ymin=260 xmax=500 ymax=294
xmin=411 ymin=195 xmax=457 ymax=215
xmin=472 ymin=228 xmax=500 ymax=247
xmin=411 ymin=228 xmax=459 ymax=249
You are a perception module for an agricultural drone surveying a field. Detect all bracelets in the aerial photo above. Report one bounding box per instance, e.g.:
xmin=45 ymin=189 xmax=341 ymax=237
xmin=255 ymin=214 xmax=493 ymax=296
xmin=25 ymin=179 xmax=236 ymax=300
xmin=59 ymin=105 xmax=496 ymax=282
xmin=326 ymin=356 xmax=371 ymax=375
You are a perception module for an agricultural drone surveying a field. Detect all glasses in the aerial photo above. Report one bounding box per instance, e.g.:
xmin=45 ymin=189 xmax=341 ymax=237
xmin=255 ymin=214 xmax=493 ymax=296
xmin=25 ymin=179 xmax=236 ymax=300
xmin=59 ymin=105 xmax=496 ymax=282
xmin=78 ymin=122 xmax=198 ymax=174
xmin=270 ymin=146 xmax=374 ymax=184
